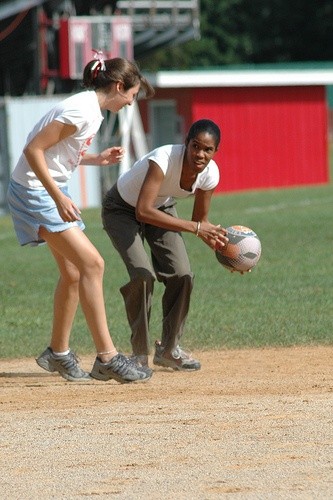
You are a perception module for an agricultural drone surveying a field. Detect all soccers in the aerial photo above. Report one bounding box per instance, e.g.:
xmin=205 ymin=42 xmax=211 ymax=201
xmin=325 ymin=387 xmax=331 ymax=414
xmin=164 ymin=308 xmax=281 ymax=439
xmin=214 ymin=224 xmax=262 ymax=273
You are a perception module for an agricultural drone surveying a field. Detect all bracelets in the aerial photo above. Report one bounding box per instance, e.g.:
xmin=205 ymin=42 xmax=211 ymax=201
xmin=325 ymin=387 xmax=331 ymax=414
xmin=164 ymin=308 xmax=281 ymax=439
xmin=195 ymin=221 xmax=201 ymax=239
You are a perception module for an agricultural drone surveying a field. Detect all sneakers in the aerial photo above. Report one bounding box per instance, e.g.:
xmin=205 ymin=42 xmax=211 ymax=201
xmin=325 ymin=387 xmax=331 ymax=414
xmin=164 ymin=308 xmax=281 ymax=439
xmin=89 ymin=353 xmax=154 ymax=382
xmin=33 ymin=347 xmax=92 ymax=381
xmin=154 ymin=338 xmax=202 ymax=372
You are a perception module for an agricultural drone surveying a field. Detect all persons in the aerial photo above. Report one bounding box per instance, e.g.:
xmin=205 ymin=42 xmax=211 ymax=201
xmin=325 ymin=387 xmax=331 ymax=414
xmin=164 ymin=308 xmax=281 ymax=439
xmin=8 ymin=50 xmax=154 ymax=383
xmin=101 ymin=118 xmax=229 ymax=377
